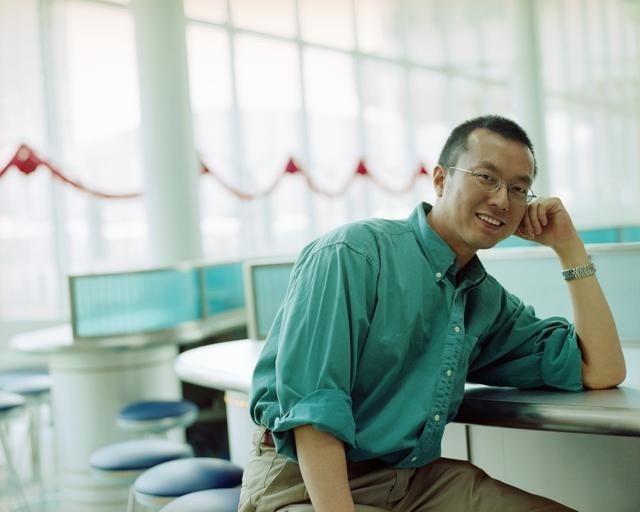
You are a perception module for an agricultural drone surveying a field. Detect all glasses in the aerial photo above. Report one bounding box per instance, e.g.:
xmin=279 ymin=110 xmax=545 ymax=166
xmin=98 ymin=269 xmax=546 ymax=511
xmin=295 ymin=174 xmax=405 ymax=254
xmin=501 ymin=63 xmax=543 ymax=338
xmin=449 ymin=167 xmax=537 ymax=204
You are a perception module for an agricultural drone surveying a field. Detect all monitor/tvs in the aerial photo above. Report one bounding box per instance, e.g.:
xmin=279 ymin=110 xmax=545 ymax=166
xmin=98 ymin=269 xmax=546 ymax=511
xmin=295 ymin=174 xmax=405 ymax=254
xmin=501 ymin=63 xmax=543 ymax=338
xmin=242 ymin=256 xmax=299 ymax=346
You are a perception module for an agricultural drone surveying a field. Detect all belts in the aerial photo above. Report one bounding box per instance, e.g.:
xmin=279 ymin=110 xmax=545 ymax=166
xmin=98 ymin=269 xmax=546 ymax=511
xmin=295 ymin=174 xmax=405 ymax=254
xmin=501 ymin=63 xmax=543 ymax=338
xmin=251 ymin=428 xmax=275 ymax=448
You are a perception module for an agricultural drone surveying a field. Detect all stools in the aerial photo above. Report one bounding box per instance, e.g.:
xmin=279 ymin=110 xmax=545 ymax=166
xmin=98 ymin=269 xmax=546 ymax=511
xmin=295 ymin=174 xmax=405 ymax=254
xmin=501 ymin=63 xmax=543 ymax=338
xmin=89 ymin=399 xmax=246 ymax=511
xmin=3 ymin=361 xmax=55 ymax=506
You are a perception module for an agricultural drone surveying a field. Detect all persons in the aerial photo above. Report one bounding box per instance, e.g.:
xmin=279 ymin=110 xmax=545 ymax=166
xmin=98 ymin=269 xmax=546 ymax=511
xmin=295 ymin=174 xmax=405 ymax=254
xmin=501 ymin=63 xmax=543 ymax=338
xmin=235 ymin=115 xmax=629 ymax=511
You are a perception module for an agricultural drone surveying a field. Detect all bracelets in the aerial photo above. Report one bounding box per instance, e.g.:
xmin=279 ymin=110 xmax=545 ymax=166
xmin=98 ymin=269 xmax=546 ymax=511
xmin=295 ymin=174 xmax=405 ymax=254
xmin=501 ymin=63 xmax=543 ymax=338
xmin=561 ymin=256 xmax=596 ymax=281
